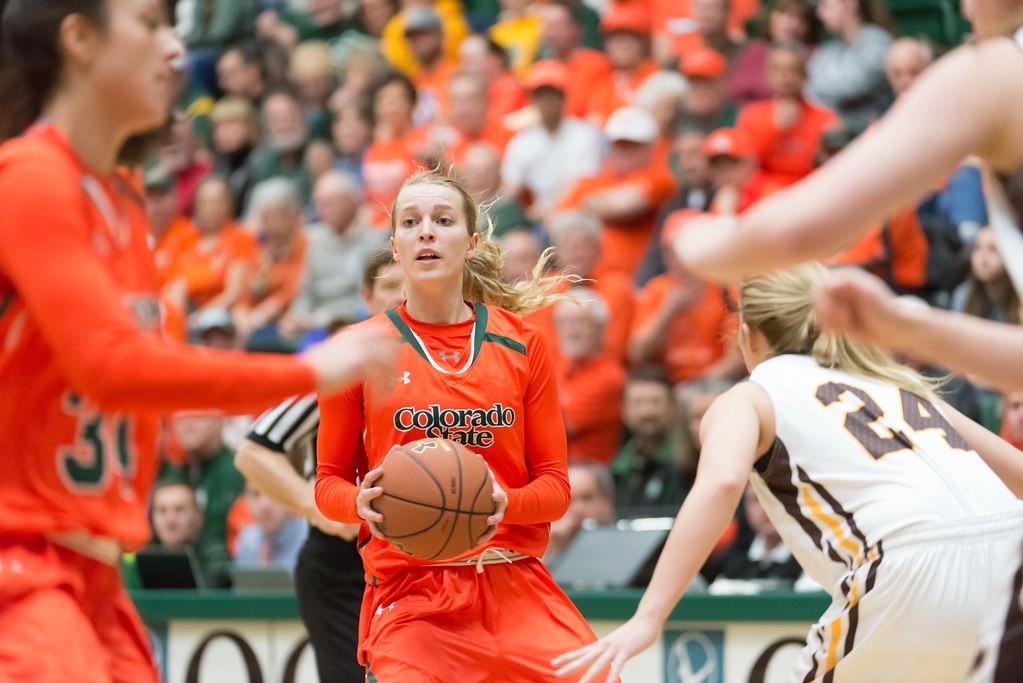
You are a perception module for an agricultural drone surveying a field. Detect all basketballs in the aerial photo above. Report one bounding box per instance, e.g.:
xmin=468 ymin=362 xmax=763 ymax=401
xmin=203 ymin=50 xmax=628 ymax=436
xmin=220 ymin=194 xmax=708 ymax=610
xmin=378 ymin=436 xmax=500 ymax=560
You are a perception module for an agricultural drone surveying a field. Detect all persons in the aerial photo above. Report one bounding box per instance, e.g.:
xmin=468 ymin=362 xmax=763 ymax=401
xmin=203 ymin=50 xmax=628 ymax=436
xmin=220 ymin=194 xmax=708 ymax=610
xmin=0 ymin=0 xmax=1023 ymax=683
